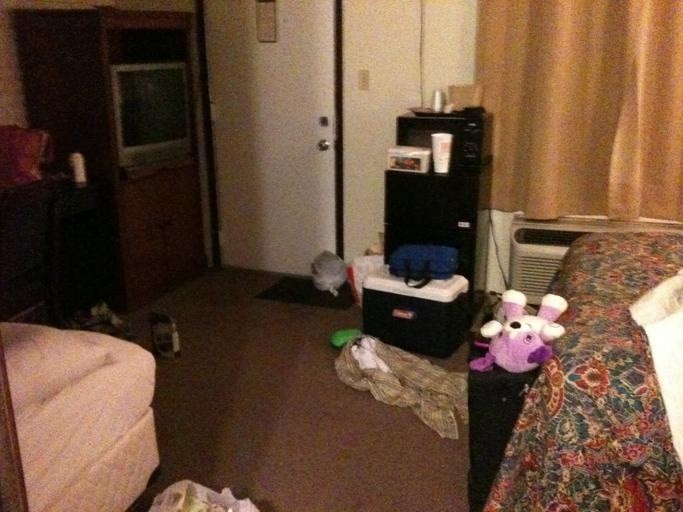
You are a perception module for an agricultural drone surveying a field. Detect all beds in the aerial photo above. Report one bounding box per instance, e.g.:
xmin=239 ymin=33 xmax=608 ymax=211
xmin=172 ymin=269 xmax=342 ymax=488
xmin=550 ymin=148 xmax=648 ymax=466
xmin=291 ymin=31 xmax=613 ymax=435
xmin=526 ymin=232 xmax=683 ymax=511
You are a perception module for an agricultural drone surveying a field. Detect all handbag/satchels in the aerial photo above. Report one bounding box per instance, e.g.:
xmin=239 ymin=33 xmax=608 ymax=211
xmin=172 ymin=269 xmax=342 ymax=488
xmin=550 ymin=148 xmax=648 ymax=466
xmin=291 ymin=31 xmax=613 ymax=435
xmin=0 ymin=125 xmax=56 ymax=192
xmin=388 ymin=244 xmax=459 ymax=288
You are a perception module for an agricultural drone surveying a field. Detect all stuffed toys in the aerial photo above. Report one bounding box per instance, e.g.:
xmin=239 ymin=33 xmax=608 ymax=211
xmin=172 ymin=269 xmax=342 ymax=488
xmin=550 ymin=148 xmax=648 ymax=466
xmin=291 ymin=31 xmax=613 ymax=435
xmin=468 ymin=289 xmax=571 ymax=374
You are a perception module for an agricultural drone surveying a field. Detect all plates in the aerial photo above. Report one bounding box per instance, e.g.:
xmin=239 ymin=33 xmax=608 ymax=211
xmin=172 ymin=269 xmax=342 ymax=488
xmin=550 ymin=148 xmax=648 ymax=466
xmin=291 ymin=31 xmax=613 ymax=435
xmin=406 ymin=106 xmax=452 ymax=117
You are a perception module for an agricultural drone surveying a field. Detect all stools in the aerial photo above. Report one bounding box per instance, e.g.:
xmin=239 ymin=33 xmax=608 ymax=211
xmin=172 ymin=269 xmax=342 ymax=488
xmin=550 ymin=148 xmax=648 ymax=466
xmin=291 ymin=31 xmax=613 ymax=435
xmin=13 ymin=328 xmax=160 ymax=511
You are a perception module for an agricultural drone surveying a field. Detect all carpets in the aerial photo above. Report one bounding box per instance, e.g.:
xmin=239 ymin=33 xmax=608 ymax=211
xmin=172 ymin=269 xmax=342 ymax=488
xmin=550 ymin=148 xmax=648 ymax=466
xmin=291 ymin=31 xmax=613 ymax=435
xmin=251 ymin=275 xmax=356 ymax=312
xmin=464 ymin=330 xmax=541 ymax=512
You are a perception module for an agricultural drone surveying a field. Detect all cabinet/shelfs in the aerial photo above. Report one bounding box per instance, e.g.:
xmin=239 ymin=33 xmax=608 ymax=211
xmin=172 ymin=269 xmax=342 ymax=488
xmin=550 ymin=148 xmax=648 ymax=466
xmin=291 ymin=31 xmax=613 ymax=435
xmin=382 ymin=110 xmax=495 ymax=328
xmin=7 ymin=5 xmax=208 ymax=317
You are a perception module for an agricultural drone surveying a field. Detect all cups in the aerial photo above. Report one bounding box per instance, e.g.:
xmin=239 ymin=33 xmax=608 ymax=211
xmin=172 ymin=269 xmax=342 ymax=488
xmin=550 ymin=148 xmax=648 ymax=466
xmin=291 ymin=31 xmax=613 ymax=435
xmin=431 ymin=89 xmax=447 ymax=113
xmin=430 ymin=131 xmax=453 ymax=173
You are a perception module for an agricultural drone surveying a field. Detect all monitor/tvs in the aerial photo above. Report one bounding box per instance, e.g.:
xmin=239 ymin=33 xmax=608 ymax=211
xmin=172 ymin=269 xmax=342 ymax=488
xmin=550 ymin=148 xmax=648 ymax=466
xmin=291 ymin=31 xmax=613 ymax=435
xmin=109 ymin=61 xmax=192 ymax=172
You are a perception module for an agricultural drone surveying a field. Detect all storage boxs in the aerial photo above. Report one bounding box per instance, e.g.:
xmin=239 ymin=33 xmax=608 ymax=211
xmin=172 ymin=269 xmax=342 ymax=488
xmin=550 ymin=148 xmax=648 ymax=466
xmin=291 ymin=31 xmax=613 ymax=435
xmin=359 ymin=265 xmax=471 ymax=359
